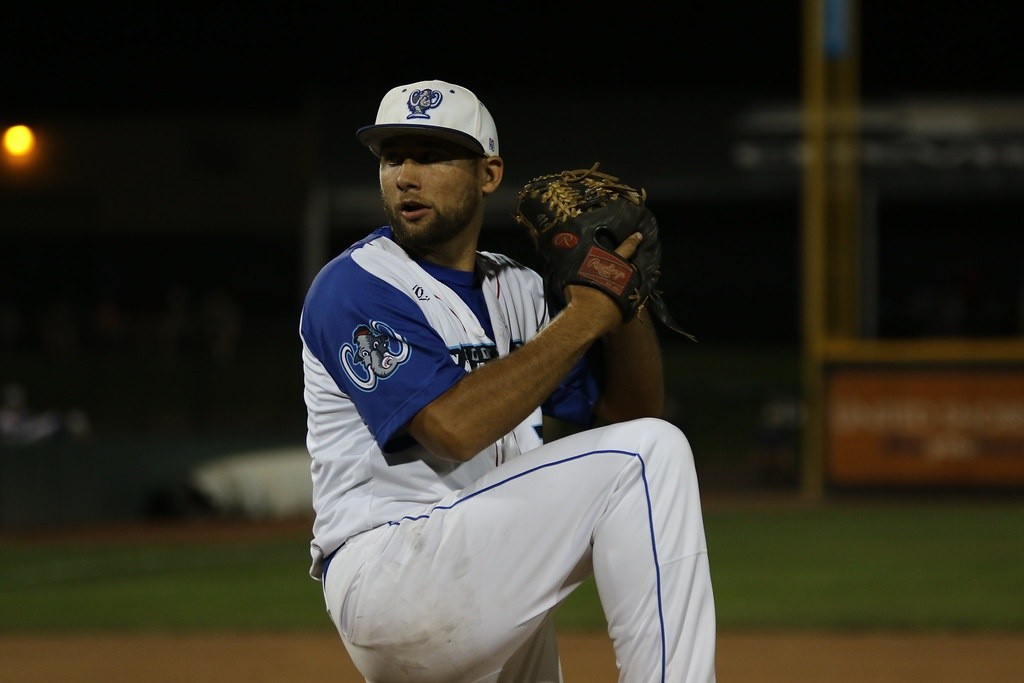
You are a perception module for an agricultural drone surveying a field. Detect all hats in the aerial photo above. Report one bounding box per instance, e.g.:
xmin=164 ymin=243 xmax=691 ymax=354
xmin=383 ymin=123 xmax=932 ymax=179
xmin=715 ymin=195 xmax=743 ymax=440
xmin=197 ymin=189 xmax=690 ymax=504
xmin=356 ymin=79 xmax=500 ymax=158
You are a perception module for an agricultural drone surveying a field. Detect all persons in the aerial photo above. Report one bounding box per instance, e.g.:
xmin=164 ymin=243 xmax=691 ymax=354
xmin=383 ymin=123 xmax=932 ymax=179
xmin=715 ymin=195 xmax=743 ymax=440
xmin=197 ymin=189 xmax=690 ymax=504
xmin=298 ymin=79 xmax=717 ymax=683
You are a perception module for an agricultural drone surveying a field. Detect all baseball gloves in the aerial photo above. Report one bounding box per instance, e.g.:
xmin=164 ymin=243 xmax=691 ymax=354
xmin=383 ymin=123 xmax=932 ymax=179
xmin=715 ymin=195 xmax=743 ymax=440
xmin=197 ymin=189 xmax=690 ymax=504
xmin=515 ymin=167 xmax=661 ymax=317
